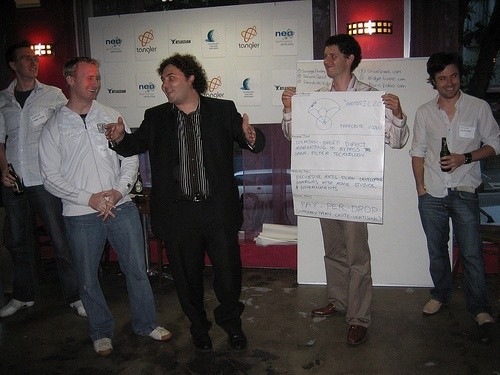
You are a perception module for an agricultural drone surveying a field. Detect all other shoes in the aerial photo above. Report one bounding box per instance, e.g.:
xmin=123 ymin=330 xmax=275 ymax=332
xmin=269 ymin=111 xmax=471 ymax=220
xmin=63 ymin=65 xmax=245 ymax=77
xmin=423 ymin=299 xmax=443 ymax=315
xmin=474 ymin=310 xmax=497 ymax=326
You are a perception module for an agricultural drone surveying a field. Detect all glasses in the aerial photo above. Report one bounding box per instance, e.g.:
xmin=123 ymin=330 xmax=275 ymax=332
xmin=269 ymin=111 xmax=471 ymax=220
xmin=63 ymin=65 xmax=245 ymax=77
xmin=15 ymin=55 xmax=38 ymax=63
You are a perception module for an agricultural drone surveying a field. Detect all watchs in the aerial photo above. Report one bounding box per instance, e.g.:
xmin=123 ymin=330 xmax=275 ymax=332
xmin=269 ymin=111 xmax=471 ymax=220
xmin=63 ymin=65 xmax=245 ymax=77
xmin=464 ymin=153 xmax=472 ymax=164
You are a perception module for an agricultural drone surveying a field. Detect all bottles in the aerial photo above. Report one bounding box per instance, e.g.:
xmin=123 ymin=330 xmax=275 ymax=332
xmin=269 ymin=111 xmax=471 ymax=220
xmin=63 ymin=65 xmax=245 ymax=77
xmin=440 ymin=137 xmax=451 ymax=172
xmin=8 ymin=163 xmax=24 ymax=194
xmin=134 ymin=167 xmax=144 ymax=195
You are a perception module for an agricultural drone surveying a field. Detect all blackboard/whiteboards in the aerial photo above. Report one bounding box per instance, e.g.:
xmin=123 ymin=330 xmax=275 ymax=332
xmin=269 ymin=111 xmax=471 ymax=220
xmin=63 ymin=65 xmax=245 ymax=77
xmin=296 ymin=57 xmax=452 ymax=287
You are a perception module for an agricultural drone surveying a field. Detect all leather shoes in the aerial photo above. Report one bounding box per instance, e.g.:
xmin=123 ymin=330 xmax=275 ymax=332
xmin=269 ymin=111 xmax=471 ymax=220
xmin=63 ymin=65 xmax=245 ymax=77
xmin=311 ymin=303 xmax=341 ymax=317
xmin=227 ymin=328 xmax=248 ymax=353
xmin=346 ymin=325 xmax=368 ymax=345
xmin=193 ymin=334 xmax=213 ymax=353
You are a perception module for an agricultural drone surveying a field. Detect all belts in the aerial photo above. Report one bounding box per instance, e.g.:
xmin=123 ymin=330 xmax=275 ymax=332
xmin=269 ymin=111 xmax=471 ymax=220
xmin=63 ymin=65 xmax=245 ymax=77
xmin=177 ymin=195 xmax=213 ymax=205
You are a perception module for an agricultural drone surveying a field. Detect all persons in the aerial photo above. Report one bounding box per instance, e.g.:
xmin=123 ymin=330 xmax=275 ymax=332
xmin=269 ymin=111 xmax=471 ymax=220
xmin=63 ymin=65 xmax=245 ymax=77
xmin=280 ymin=33 xmax=410 ymax=345
xmin=410 ymin=50 xmax=500 ymax=342
xmin=38 ymin=55 xmax=172 ymax=353
xmin=0 ymin=38 xmax=88 ymax=318
xmin=104 ymin=53 xmax=266 ymax=353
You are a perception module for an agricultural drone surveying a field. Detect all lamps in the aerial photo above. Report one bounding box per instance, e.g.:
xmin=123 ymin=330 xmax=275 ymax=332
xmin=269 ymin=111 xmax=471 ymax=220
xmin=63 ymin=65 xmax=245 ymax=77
xmin=30 ymin=44 xmax=55 ymax=56
xmin=347 ymin=21 xmax=393 ymax=36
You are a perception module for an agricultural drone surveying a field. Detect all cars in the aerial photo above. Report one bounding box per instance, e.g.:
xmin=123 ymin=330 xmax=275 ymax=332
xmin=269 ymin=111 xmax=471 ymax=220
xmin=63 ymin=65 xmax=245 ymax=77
xmin=233 ymin=168 xmax=294 ymax=210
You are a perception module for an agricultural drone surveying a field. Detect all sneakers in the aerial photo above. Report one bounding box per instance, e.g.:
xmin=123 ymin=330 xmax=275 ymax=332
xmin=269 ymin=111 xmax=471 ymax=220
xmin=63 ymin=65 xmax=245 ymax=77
xmin=0 ymin=298 xmax=35 ymax=318
xmin=94 ymin=337 xmax=113 ymax=356
xmin=149 ymin=326 xmax=172 ymax=341
xmin=69 ymin=299 xmax=88 ymax=318
